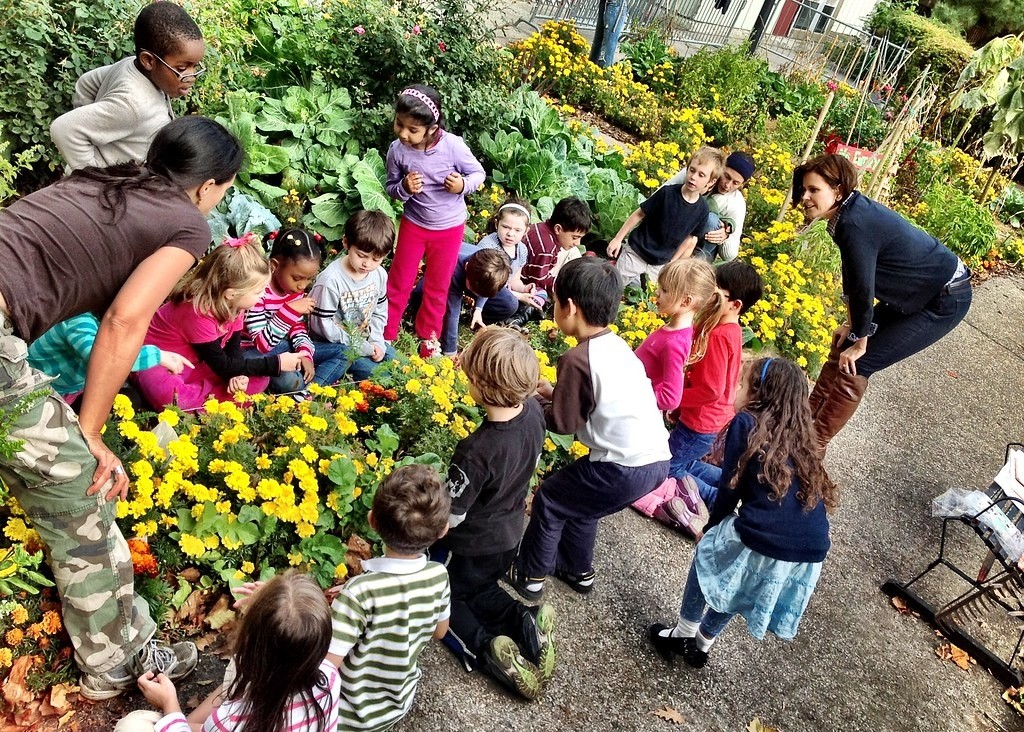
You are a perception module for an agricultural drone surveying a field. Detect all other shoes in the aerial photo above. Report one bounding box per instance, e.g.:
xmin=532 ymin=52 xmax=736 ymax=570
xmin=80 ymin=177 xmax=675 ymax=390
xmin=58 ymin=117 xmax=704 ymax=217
xmin=684 ymin=648 xmax=708 ymax=669
xmin=650 ymin=623 xmax=696 ymax=655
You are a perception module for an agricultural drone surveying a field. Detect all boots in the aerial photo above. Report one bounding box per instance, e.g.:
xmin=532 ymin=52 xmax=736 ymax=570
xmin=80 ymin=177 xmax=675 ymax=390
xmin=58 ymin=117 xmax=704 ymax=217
xmin=505 ymin=305 xmax=534 ymax=328
xmin=810 ymin=370 xmax=868 ymax=462
xmin=808 ymin=346 xmax=845 ymax=420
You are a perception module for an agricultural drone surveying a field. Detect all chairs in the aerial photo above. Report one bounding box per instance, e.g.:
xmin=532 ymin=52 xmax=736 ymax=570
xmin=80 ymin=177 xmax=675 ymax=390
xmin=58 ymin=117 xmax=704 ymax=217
xmin=881 ymin=442 xmax=1024 ymax=686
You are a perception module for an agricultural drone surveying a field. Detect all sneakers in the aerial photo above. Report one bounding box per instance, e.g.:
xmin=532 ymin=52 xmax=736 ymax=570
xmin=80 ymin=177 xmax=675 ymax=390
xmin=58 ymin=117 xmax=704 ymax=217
xmin=516 ymin=601 xmax=557 ymax=680
xmin=477 ymin=634 xmax=543 ymax=699
xmin=79 ymin=632 xmax=197 ymax=700
xmin=419 ymin=339 xmax=440 ymax=361
xmin=675 ymin=475 xmax=709 ymax=524
xmin=654 ymin=496 xmax=707 ymax=539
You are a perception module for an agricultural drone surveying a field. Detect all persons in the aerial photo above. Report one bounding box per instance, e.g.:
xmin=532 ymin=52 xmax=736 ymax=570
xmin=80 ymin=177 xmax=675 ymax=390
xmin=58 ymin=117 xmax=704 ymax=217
xmin=501 ymin=256 xmax=672 ymax=601
xmin=49 ymin=1 xmax=207 ymax=178
xmin=137 ymin=231 xmax=309 ymax=418
xmin=630 ymin=257 xmax=727 ymax=542
xmin=410 ymin=240 xmax=513 ymax=358
xmin=29 ymin=311 xmax=195 ymax=406
xmin=647 ymin=357 xmax=840 ymax=670
xmin=0 ymin=115 xmax=246 ymax=700
xmin=655 ymin=152 xmax=756 ymax=265
xmin=520 ymin=198 xmax=592 ymax=298
xmin=383 ymin=84 xmax=486 ymax=358
xmin=325 ymin=465 xmax=452 ymax=732
xmin=240 ymin=226 xmax=354 ymax=403
xmin=424 ymin=325 xmax=557 ymax=700
xmin=606 ymin=146 xmax=723 ymax=287
xmin=668 ymin=261 xmax=764 ymax=510
xmin=306 ymin=210 xmax=413 ymax=386
xmin=791 ymin=154 xmax=972 ymax=459
xmin=115 ymin=568 xmax=341 ymax=732
xmin=477 ymin=198 xmax=542 ymax=326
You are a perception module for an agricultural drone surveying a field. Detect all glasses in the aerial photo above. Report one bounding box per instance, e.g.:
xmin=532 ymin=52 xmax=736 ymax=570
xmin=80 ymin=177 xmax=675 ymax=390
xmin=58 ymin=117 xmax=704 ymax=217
xmin=140 ymin=48 xmax=207 ymax=83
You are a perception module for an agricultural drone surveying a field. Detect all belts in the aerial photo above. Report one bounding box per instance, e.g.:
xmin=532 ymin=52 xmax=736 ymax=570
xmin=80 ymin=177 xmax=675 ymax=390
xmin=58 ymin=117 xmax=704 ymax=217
xmin=941 ymin=269 xmax=975 ymax=290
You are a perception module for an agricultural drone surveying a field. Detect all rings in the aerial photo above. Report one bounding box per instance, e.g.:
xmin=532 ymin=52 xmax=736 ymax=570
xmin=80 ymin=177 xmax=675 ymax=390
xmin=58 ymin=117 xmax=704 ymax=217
xmin=114 ymin=465 xmax=125 ymax=475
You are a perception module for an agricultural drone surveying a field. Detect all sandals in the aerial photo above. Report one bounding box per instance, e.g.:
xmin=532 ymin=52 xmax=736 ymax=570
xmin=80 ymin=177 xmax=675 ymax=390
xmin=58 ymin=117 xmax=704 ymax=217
xmin=553 ymin=564 xmax=595 ymax=593
xmin=500 ymin=555 xmax=545 ymax=600
